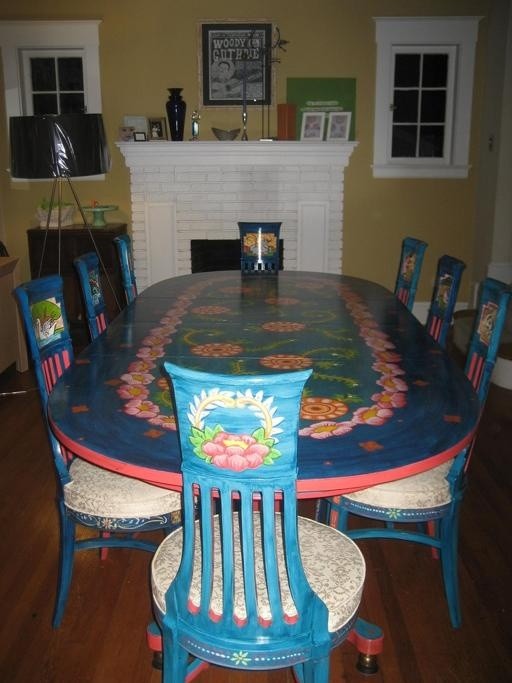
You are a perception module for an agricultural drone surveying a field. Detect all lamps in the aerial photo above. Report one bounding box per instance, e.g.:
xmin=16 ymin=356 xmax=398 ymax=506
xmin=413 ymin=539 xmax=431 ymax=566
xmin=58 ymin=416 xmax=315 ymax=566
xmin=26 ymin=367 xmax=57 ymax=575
xmin=7 ymin=114 xmax=125 ymax=314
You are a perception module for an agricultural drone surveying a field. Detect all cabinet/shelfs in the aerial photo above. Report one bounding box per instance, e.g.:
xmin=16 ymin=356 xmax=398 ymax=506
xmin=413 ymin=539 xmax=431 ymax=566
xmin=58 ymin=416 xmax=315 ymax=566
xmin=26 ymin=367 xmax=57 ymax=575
xmin=25 ymin=222 xmax=127 ymax=360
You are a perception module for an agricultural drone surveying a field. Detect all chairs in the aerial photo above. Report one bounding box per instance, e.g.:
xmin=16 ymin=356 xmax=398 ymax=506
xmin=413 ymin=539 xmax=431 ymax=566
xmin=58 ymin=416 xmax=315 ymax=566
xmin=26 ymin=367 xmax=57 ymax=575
xmin=398 ymin=238 xmax=429 ymax=308
xmin=422 ymin=252 xmax=464 ymax=346
xmin=13 ymin=276 xmax=203 ymax=626
xmin=115 ymin=239 xmax=138 ymax=301
xmin=71 ymin=251 xmax=108 ymax=341
xmin=314 ymin=278 xmax=506 ymax=631
xmin=147 ymin=357 xmax=367 ymax=681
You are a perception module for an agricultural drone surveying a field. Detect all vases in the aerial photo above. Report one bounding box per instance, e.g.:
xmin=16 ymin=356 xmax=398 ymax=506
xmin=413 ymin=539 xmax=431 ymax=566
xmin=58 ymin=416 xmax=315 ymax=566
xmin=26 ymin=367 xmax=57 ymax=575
xmin=166 ymin=86 xmax=187 ymax=139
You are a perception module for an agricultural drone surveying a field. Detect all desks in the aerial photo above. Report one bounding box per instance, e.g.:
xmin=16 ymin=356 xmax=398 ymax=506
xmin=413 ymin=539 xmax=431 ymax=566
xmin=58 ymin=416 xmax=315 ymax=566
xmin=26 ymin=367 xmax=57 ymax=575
xmin=54 ymin=269 xmax=484 ymax=673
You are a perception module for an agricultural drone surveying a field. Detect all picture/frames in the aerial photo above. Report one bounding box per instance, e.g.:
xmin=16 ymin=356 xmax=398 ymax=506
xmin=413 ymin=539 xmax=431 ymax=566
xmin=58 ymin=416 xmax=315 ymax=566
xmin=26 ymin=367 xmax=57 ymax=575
xmin=194 ymin=17 xmax=278 ymax=113
xmin=299 ymin=112 xmax=352 ymax=141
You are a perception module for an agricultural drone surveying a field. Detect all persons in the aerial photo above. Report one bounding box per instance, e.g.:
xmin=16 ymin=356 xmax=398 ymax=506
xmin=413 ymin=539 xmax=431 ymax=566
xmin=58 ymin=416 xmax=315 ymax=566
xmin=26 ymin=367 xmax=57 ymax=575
xmin=118 ymin=125 xmax=135 ymax=140
xmin=210 ymin=58 xmax=241 ymax=98
